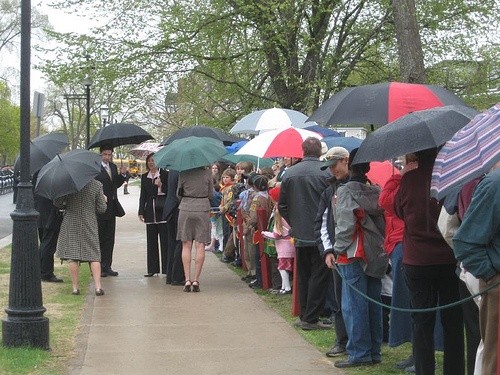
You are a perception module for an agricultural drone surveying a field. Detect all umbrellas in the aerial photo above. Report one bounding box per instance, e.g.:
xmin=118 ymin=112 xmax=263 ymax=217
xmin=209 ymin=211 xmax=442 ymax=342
xmin=14 ymin=80 xmax=500 ymax=205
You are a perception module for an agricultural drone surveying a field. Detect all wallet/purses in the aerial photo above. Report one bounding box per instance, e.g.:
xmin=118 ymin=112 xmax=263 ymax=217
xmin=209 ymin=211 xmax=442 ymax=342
xmin=179 ymin=187 xmax=183 ymax=195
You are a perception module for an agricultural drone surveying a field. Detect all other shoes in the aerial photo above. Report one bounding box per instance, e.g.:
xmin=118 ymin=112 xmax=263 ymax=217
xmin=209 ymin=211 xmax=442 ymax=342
xmin=220 ymin=254 xmax=258 ymax=288
xmin=101 ymin=270 xmax=118 ymax=277
xmin=43 ymin=272 xmax=63 ymax=282
xmin=276 ymin=288 xmax=291 ymax=295
xmin=205 ymin=245 xmax=215 ymax=252
xmin=72 ymin=289 xmax=80 ymax=295
xmin=334 ymin=359 xmax=383 ymax=367
xmin=95 ymin=288 xmax=104 ymax=295
xmin=396 ymin=356 xmax=438 ymax=372
xmin=296 ymin=318 xmax=333 ymax=330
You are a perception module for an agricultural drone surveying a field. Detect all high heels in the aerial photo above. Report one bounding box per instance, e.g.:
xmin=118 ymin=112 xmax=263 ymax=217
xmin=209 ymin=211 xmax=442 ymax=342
xmin=144 ymin=272 xmax=159 ymax=277
xmin=183 ymin=280 xmax=192 ymax=292
xmin=191 ymin=280 xmax=200 ymax=292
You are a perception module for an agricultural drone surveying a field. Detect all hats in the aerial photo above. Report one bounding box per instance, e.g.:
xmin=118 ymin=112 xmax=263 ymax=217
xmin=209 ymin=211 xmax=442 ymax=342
xmin=320 ymin=147 xmax=350 ymax=170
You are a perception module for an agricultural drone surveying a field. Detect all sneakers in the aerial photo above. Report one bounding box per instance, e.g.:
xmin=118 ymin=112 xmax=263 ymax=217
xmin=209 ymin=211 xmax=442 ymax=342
xmin=326 ymin=344 xmax=347 ymax=357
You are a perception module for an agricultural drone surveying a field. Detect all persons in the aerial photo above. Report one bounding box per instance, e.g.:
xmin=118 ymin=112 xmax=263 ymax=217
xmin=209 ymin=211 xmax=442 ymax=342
xmin=32 ymin=169 xmax=64 ymax=283
xmin=0 ymin=165 xmax=15 ymax=195
xmin=94 ymin=144 xmax=125 ymax=276
xmin=54 ymin=179 xmax=107 ymax=296
xmin=138 ymin=136 xmax=500 ymax=375
xmin=121 ymin=167 xmax=131 ymax=194
